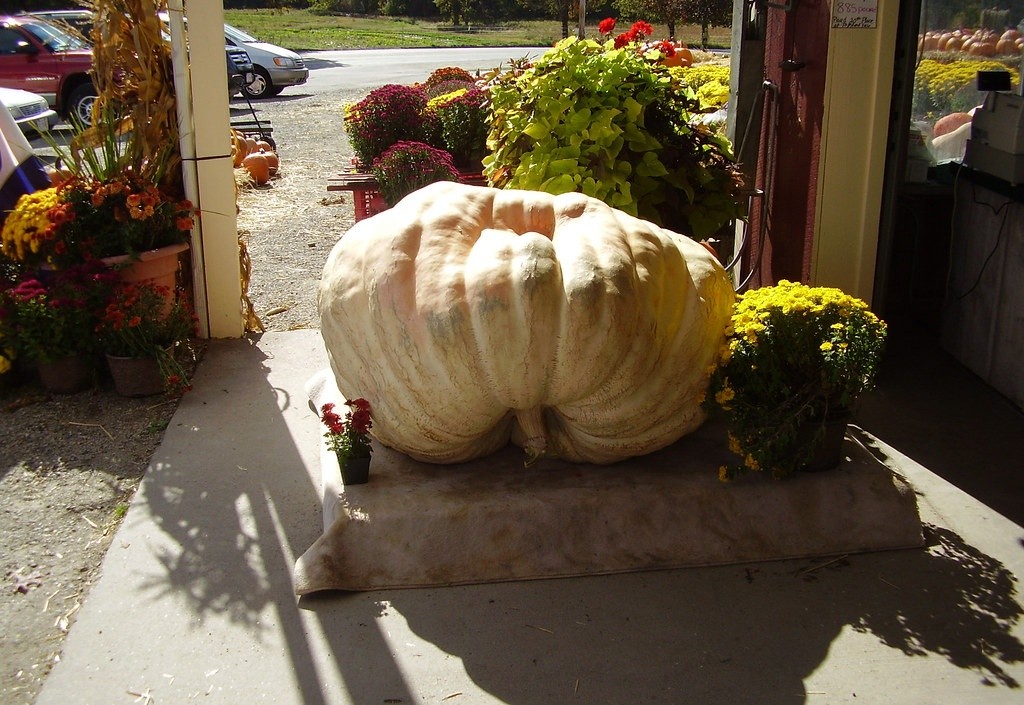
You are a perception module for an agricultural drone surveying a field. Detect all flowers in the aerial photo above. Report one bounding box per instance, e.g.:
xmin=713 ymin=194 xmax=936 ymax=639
xmin=696 ymin=279 xmax=888 ymax=483
xmin=308 ymin=398 xmax=374 ymax=458
xmin=0 ymin=98 xmax=200 ymax=396
xmin=343 ymin=17 xmax=753 ymax=266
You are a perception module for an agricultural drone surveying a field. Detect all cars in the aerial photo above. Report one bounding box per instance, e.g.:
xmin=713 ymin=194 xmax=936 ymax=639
xmin=30 ymin=10 xmax=255 ymax=100
xmin=0 ymin=14 xmax=161 ymax=132
xmin=159 ymin=10 xmax=308 ymax=99
xmin=0 ymin=85 xmax=59 ymax=140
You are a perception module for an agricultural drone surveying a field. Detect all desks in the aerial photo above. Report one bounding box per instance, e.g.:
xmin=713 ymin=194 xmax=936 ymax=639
xmin=940 ymin=162 xmax=1023 ymax=416
xmin=327 ymin=165 xmax=489 ymax=224
xmin=886 ymin=179 xmax=955 ymax=320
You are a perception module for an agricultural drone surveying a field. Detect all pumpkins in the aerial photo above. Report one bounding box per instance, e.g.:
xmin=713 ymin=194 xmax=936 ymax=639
xmin=918 ymin=27 xmax=1024 ymax=56
xmin=640 ymin=40 xmax=693 ymax=66
xmin=231 ymin=131 xmax=278 ymax=184
xmin=934 ymin=111 xmax=972 ymax=137
xmin=317 ymin=181 xmax=737 ymax=459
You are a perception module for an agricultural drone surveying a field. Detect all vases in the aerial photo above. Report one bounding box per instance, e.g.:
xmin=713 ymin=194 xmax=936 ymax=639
xmin=101 ymin=240 xmax=189 ymax=322
xmin=40 ymin=352 xmax=92 ymax=393
xmin=107 ymin=344 xmax=175 ymax=397
xmin=337 ymin=458 xmax=370 ymax=486
xmin=790 ymin=414 xmax=853 ymax=471
